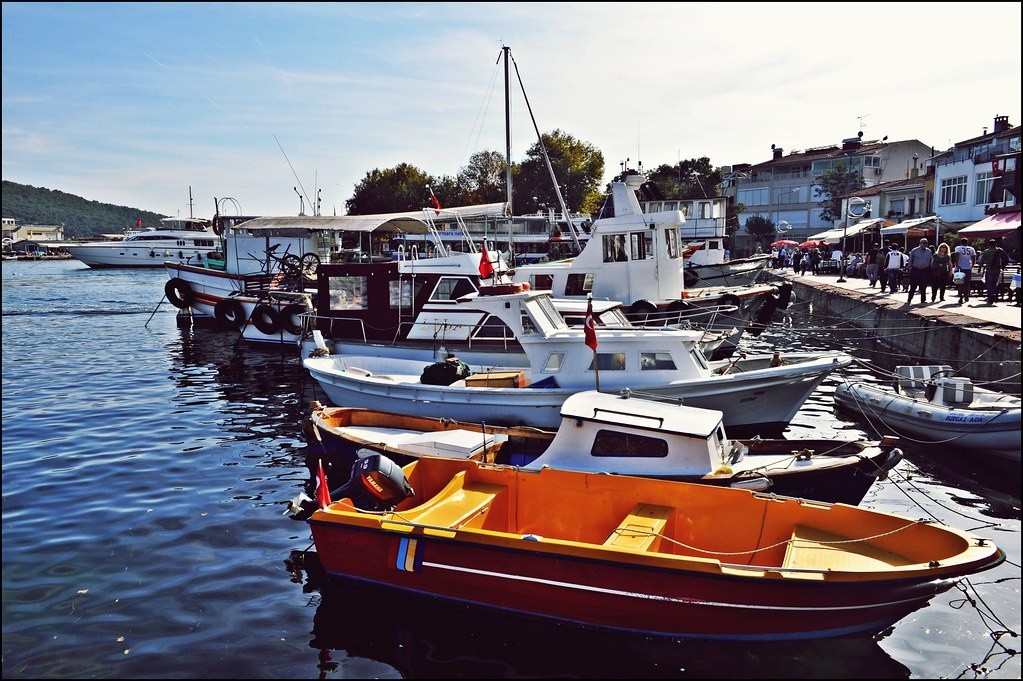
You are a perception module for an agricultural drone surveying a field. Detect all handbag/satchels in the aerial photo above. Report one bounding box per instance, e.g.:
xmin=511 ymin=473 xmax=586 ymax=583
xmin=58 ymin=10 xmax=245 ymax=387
xmin=940 ymin=264 xmax=950 ymax=275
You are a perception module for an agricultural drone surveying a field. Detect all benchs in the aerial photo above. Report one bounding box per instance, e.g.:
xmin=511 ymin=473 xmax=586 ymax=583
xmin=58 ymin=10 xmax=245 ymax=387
xmin=970 ymin=264 xmax=1022 ymax=299
xmin=407 ymin=482 xmax=509 ymax=528
xmin=601 ymin=503 xmax=676 ymax=552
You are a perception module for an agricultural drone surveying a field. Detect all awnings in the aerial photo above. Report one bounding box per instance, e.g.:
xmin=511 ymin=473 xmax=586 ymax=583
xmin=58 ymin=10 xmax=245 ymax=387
xmin=957 ymin=212 xmax=1022 ymax=238
xmin=824 ymin=219 xmax=881 ymax=255
xmin=880 ymin=214 xmax=939 ymax=252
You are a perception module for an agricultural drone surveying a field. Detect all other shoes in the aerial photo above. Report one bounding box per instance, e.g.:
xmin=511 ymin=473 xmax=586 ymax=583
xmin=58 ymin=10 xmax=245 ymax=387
xmin=890 ymin=288 xmax=898 ymax=294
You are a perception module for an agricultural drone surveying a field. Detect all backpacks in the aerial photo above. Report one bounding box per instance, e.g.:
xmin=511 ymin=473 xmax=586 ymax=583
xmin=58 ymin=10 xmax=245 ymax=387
xmin=876 ymin=253 xmax=885 ymax=265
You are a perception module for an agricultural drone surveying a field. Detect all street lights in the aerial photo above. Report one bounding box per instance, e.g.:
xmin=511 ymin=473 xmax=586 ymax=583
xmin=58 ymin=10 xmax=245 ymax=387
xmin=777 ymin=188 xmax=800 ymax=240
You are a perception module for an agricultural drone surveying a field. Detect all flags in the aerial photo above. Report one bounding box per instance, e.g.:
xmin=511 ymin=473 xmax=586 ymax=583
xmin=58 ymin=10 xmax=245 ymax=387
xmin=584 ymin=305 xmax=597 ymax=352
xmin=427 ymin=190 xmax=441 ymax=215
xmin=479 ymin=245 xmax=495 ymax=279
xmin=136 ymin=219 xmax=142 ymax=227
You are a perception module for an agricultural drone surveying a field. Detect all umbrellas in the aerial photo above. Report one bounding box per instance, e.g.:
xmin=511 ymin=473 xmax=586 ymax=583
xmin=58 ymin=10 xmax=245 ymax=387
xmin=799 ymin=239 xmax=832 ymax=247
xmin=770 ymin=240 xmax=799 ymax=249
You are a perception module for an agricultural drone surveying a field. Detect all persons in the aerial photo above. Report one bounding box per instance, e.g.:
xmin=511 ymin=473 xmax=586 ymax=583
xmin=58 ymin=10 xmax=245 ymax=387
xmin=771 ymin=245 xmax=1020 ymax=276
xmin=865 ymin=243 xmax=879 ymax=287
xmin=884 ymin=243 xmax=903 ymax=293
xmin=954 ymin=237 xmax=976 ymax=303
xmin=977 ymin=239 xmax=1009 ymax=305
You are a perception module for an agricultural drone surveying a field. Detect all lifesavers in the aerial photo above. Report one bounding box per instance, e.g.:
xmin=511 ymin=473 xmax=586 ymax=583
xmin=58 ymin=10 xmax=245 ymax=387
xmin=251 ymin=305 xmax=279 ymax=335
xmin=666 ymin=301 xmax=691 ymax=324
xmin=628 ymin=299 xmax=658 ymax=326
xmin=279 ymin=305 xmax=309 ymax=335
xmin=684 ymin=268 xmax=698 ymax=288
xmin=522 ymin=282 xmax=530 ymax=292
xmin=214 ymin=300 xmax=246 ymax=329
xmin=478 ymin=283 xmax=523 ymax=297
xmin=164 ymin=278 xmax=196 ymax=308
xmin=717 ymin=294 xmax=741 ymax=316
xmin=212 ymin=214 xmax=224 ymax=235
xmin=779 ymin=281 xmax=793 ymax=310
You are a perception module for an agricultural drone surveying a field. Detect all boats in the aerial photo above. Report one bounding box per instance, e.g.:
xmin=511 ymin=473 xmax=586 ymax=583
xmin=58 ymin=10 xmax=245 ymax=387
xmin=298 ymin=292 xmax=905 ymax=506
xmin=60 ymin=187 xmax=227 ymax=269
xmin=282 ymin=448 xmax=1007 ymax=644
xmin=165 ymin=46 xmax=855 ymax=439
xmin=834 ymin=364 xmax=1023 ymax=463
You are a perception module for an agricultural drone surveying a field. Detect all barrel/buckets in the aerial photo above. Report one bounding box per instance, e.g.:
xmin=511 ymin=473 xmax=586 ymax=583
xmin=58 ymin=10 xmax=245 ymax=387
xmin=953 ymin=268 xmax=965 ymax=285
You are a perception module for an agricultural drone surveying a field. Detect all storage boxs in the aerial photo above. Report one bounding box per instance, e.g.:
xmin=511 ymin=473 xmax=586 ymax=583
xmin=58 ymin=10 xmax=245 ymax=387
xmin=392 ymin=251 xmax=409 ymax=261
